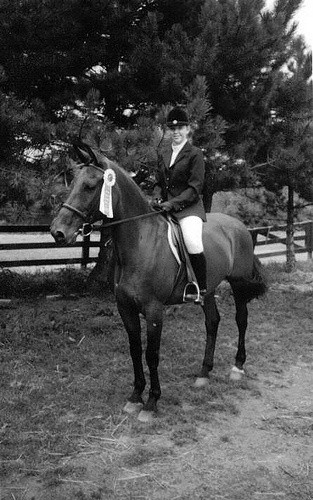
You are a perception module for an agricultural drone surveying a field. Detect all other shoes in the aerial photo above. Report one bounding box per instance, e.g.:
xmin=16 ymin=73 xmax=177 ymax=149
xmin=186 ymin=294 xmax=205 ymax=306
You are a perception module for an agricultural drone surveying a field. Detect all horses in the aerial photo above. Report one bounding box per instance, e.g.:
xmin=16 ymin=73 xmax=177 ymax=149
xmin=50 ymin=143 xmax=271 ymax=423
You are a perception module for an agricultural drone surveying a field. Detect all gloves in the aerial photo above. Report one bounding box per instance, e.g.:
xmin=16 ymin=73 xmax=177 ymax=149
xmin=152 ymin=201 xmax=173 ymax=211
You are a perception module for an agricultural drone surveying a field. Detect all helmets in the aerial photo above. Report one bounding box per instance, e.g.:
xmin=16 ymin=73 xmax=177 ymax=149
xmin=167 ymin=109 xmax=189 ymax=126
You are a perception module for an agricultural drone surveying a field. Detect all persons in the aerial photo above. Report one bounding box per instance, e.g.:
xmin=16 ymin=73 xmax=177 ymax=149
xmin=149 ymin=107 xmax=210 ymax=306
